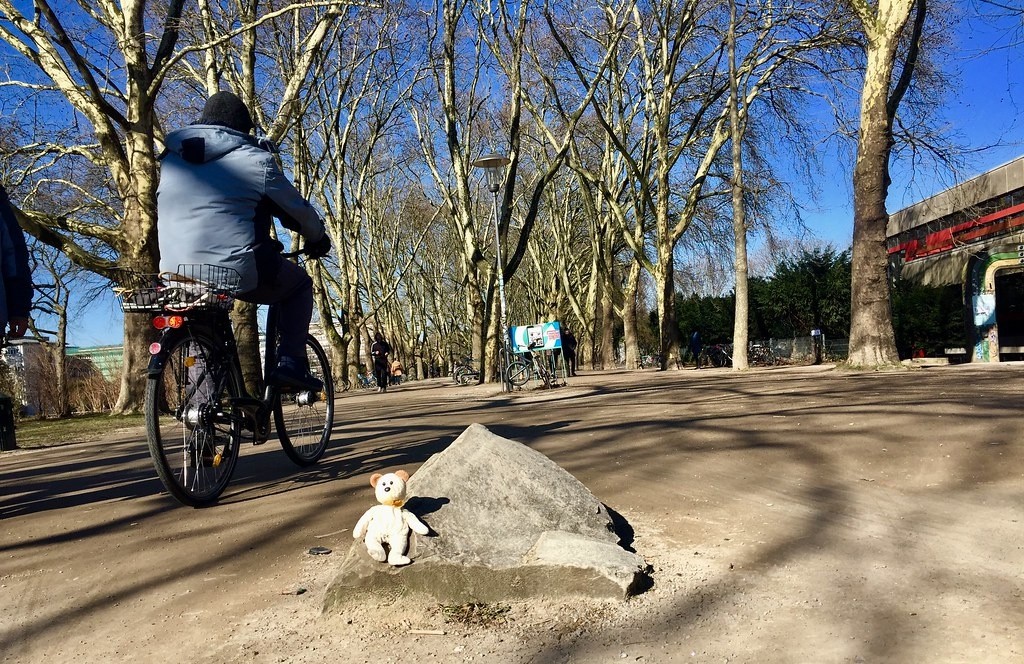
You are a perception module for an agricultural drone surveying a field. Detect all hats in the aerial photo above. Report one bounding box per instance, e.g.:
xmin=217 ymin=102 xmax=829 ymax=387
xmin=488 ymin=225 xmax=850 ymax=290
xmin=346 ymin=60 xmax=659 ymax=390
xmin=202 ymin=91 xmax=251 ymax=132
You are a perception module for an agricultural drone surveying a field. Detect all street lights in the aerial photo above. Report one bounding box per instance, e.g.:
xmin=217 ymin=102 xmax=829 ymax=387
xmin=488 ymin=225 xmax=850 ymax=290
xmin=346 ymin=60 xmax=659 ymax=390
xmin=472 ymin=152 xmax=512 ymax=392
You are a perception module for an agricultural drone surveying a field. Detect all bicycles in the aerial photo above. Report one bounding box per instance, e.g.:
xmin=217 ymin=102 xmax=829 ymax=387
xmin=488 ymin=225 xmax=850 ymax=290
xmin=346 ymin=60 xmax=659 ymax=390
xmin=320 ymin=376 xmax=351 ymax=393
xmin=108 ymin=247 xmax=335 ymax=509
xmin=691 ymin=340 xmax=783 ymax=366
xmin=516 ymin=338 xmax=550 ymax=387
xmin=453 ymin=357 xmax=475 ymax=386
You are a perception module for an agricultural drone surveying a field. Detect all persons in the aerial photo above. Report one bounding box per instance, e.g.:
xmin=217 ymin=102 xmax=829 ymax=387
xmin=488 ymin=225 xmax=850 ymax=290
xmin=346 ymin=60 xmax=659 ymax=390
xmin=546 ymin=320 xmax=577 ymax=378
xmin=688 ymin=326 xmax=701 ymax=369
xmin=157 ymin=92 xmax=332 ymax=490
xmin=429 ymin=364 xmax=434 ymax=378
xmin=358 ymin=332 xmax=406 ymax=393
xmin=0 ymin=183 xmax=34 ymax=350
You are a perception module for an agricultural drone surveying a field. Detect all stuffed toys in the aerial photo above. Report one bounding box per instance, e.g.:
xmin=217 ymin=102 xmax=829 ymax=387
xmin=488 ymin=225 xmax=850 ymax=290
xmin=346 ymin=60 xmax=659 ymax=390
xmin=353 ymin=471 xmax=429 ymax=565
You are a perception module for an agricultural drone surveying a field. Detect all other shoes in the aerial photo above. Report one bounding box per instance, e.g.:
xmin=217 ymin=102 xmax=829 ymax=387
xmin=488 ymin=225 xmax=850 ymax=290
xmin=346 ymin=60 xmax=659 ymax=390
xmin=273 ymin=356 xmax=323 ymax=392
xmin=572 ymin=373 xmax=577 ymax=376
xmin=191 ymin=448 xmax=236 ymax=467
xmin=567 ymin=374 xmax=570 ymax=377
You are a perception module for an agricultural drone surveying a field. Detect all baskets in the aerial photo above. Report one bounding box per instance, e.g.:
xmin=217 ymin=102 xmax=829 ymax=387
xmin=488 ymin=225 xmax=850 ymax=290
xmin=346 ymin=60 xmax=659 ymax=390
xmin=106 ymin=261 xmax=242 ymax=312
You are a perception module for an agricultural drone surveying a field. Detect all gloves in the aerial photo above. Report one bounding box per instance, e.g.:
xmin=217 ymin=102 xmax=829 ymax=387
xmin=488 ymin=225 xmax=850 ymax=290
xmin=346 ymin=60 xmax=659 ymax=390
xmin=305 ymin=234 xmax=331 ymax=258
